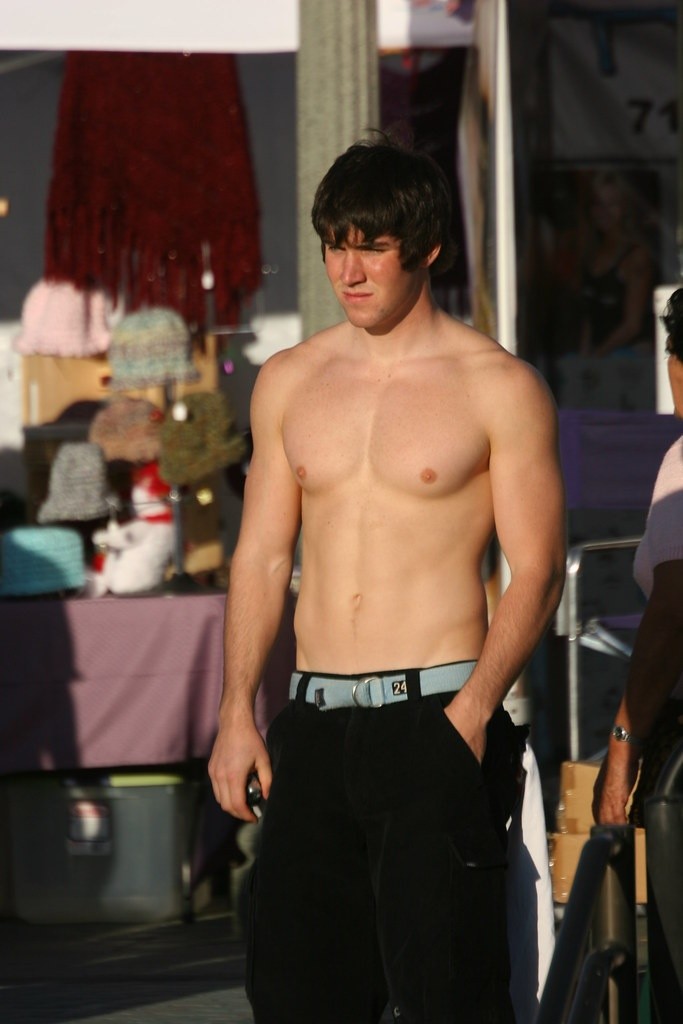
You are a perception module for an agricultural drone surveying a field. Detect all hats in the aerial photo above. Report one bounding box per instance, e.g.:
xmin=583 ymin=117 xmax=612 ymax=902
xmin=181 ymin=490 xmax=245 ymax=574
xmin=89 ymin=395 xmax=166 ymax=462
xmin=158 ymin=390 xmax=248 ymax=488
xmin=37 ymin=442 xmax=122 ymax=524
xmin=106 ymin=303 xmax=201 ymax=392
xmin=12 ymin=278 xmax=113 ymax=357
xmin=0 ymin=526 xmax=84 ymax=595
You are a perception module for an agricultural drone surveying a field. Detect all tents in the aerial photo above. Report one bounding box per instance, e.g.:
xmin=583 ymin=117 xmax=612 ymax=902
xmin=1 ymin=0 xmax=476 ymax=144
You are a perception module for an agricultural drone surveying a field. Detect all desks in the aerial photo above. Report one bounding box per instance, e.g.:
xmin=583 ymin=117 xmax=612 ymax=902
xmin=0 ymin=595 xmax=296 ymax=774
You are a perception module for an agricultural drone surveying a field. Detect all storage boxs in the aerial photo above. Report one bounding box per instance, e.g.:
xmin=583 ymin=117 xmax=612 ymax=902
xmin=4 ymin=775 xmax=194 ymax=925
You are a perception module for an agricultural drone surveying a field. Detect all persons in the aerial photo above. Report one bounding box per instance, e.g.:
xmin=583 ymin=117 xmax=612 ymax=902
xmin=208 ymin=141 xmax=567 ymax=1024
xmin=590 ymin=288 xmax=683 ymax=828
xmin=576 ymin=179 xmax=654 ymax=411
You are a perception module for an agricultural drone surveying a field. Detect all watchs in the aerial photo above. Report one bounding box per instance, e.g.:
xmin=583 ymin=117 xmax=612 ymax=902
xmin=610 ymin=725 xmax=644 ymax=746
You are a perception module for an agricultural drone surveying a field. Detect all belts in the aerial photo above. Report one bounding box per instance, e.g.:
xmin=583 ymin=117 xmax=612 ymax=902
xmin=287 ymin=663 xmax=476 ymax=712
